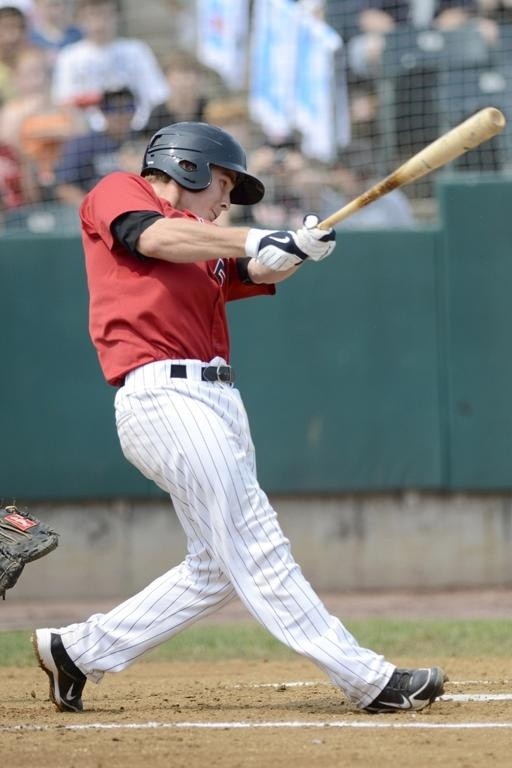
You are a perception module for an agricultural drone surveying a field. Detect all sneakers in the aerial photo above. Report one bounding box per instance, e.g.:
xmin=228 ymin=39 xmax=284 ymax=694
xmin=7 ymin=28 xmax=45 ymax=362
xmin=366 ymin=666 xmax=450 ymax=714
xmin=32 ymin=626 xmax=86 ymax=710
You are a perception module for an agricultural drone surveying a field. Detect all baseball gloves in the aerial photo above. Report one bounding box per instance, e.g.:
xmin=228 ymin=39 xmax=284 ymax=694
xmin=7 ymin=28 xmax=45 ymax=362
xmin=0 ymin=506 xmax=58 ymax=596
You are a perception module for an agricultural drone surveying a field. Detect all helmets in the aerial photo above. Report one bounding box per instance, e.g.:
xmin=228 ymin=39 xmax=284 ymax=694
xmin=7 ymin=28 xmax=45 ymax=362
xmin=142 ymin=120 xmax=265 ymax=205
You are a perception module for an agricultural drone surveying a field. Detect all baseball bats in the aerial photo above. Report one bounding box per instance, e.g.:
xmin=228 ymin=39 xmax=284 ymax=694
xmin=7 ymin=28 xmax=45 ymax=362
xmin=316 ymin=106 xmax=506 ymax=228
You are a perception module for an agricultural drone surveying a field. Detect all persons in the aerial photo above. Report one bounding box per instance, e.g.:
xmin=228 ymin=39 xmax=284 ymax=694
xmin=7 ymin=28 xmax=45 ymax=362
xmin=0 ymin=2 xmax=512 ymax=227
xmin=34 ymin=122 xmax=450 ymax=712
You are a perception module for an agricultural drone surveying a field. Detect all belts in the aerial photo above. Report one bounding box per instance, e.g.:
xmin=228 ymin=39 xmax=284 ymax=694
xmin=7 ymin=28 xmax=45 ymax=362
xmin=173 ymin=365 xmax=231 ymax=383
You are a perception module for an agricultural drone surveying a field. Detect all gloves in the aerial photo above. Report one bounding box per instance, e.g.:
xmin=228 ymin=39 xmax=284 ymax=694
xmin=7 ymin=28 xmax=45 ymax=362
xmin=297 ymin=214 xmax=336 ymax=264
xmin=245 ymin=228 xmax=307 ymax=271
